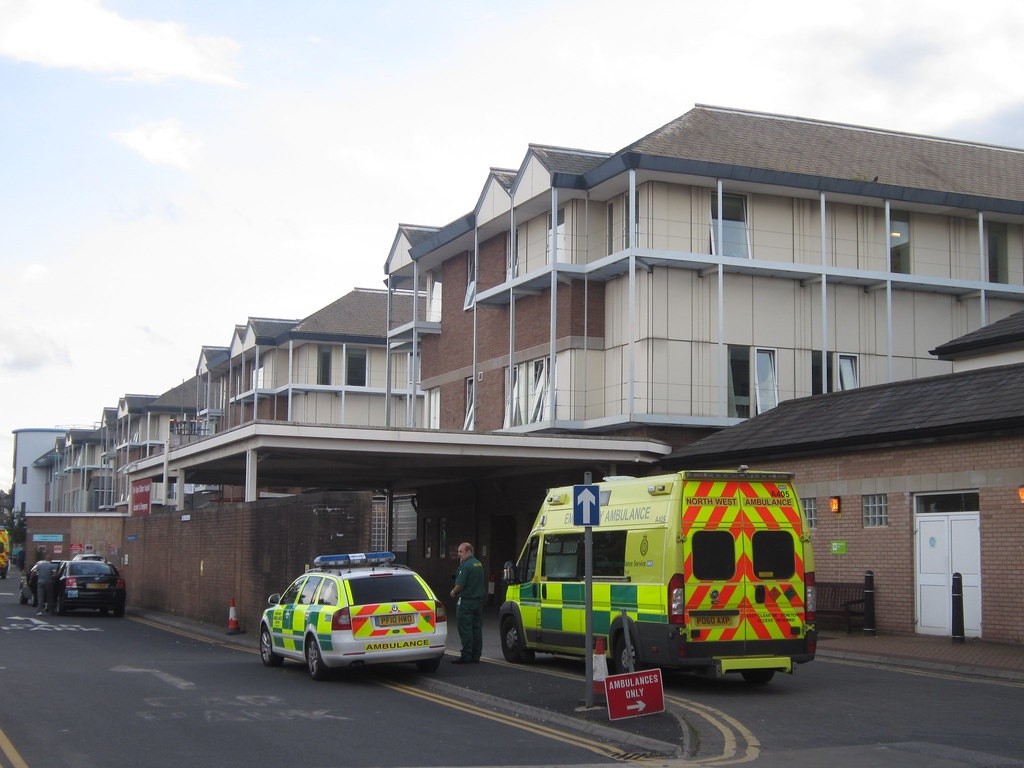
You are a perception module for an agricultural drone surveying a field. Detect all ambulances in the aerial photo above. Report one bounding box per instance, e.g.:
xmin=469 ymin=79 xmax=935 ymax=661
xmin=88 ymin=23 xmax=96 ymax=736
xmin=259 ymin=550 xmax=446 ymax=680
xmin=499 ymin=465 xmax=817 ymax=684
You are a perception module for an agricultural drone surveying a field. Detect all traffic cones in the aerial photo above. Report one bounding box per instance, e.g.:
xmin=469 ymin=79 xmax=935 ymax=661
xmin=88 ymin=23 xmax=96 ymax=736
xmin=578 ymin=636 xmax=609 ymax=707
xmin=225 ymin=599 xmax=245 ymax=636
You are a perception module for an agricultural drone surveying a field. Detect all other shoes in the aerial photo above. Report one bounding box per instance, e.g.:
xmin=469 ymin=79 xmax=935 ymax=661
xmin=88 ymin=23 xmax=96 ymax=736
xmin=36 ymin=612 xmax=43 ymax=615
xmin=451 ymin=657 xmax=480 ymax=664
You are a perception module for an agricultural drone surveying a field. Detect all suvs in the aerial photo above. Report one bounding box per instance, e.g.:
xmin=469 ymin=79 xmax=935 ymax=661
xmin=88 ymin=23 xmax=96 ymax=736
xmin=26 ymin=560 xmax=125 ymax=616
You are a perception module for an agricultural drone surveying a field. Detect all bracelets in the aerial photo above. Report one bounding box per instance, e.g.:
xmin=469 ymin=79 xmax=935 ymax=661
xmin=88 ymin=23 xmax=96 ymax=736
xmin=452 ymin=590 xmax=455 ymax=595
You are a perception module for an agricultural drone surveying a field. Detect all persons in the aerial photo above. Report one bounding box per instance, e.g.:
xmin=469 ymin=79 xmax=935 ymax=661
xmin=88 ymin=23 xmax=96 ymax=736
xmin=29 ymin=555 xmax=58 ymax=616
xmin=450 ymin=544 xmax=484 ymax=664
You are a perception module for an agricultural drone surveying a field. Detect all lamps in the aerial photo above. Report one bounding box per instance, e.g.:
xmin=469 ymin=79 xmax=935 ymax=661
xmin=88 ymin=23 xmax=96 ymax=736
xmin=828 ymin=496 xmax=840 ymax=514
xmin=1017 ymin=485 xmax=1024 ymax=504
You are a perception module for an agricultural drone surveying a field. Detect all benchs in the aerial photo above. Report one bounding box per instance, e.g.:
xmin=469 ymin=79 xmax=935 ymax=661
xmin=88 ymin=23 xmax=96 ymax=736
xmin=815 ymin=581 xmax=866 ymax=634
